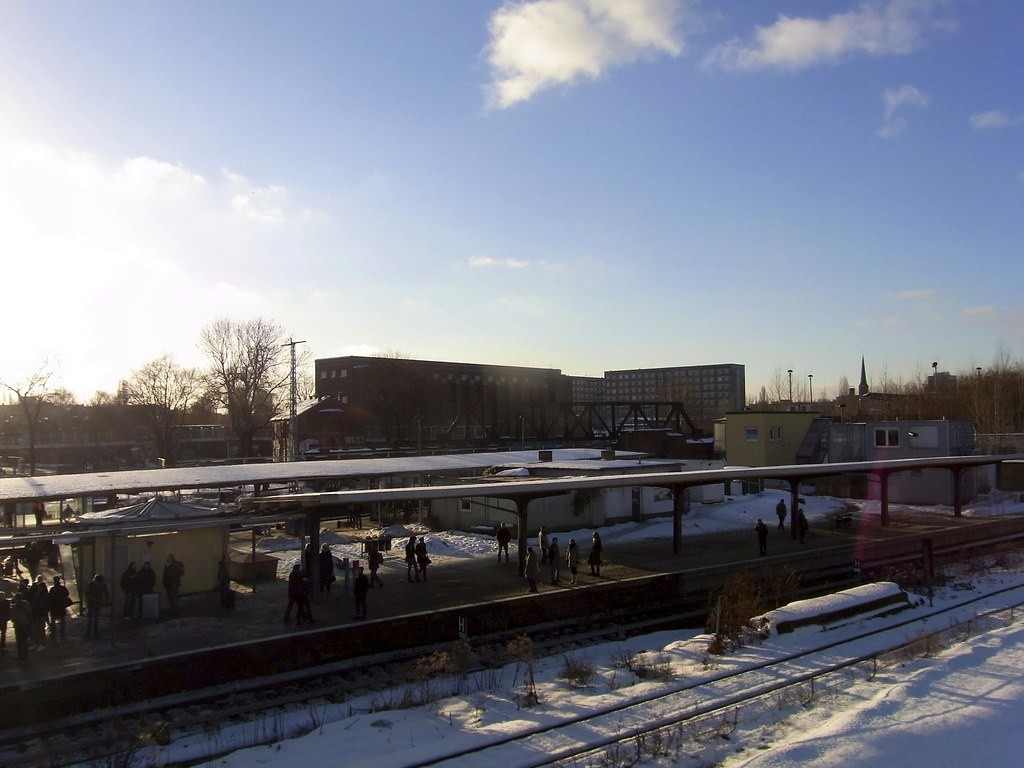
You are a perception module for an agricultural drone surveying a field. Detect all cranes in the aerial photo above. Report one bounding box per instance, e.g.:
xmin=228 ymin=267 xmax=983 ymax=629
xmin=280 ymin=336 xmax=308 ymax=494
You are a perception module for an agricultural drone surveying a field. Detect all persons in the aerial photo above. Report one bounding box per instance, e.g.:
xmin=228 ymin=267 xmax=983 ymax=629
xmin=414 ymin=537 xmax=427 ymax=582
xmin=319 ymin=542 xmax=334 ymax=593
xmin=1 ymin=553 xmax=22 ymax=574
xmin=262 ymin=509 xmax=273 ymax=537
xmin=587 ymin=532 xmax=604 ymax=576
xmin=549 ymin=537 xmax=562 ymax=586
xmin=538 ymin=525 xmax=549 ymax=565
xmin=0 ymin=575 xmax=69 ymax=660
xmin=283 ymin=564 xmax=308 ymax=623
xmin=353 ymin=566 xmax=369 ymax=620
xmin=301 ymin=543 xmax=314 ymax=579
xmin=31 ymin=502 xmax=44 ymax=527
xmin=296 ymin=570 xmax=316 ymax=624
xmin=523 ymin=547 xmax=541 ymax=592
xmin=566 ymin=538 xmax=580 ymax=584
xmin=367 ymin=543 xmax=384 ymax=589
xmin=217 ymin=553 xmax=231 ymax=605
xmin=355 ymin=513 xmax=362 ymax=530
xmin=162 ymin=554 xmax=179 ymax=615
xmin=2 ymin=504 xmax=16 ymax=526
xmin=20 ymin=542 xmax=43 ymax=581
xmin=754 ymin=499 xmax=809 ymax=556
xmin=137 ymin=561 xmax=156 ymax=613
xmin=83 ymin=574 xmax=110 ymax=637
xmin=496 ymin=522 xmax=511 ymax=563
xmin=119 ymin=561 xmax=142 ymax=620
xmin=405 ymin=535 xmax=422 ymax=584
xmin=402 ymin=499 xmax=418 ymax=522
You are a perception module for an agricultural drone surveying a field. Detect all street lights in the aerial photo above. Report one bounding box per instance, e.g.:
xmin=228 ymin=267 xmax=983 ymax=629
xmin=808 ymin=374 xmax=813 ymax=405
xmin=788 ymin=370 xmax=794 ymax=400
xmin=932 ymin=361 xmax=938 ymax=388
xmin=837 ymin=404 xmax=847 ymax=425
